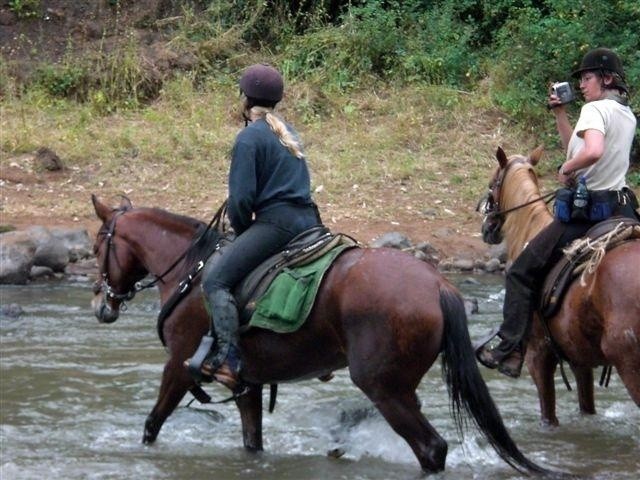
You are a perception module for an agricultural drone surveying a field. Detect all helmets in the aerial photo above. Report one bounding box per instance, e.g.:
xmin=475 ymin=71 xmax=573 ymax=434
xmin=571 ymin=48 xmax=623 ymax=76
xmin=240 ymin=62 xmax=283 ymax=101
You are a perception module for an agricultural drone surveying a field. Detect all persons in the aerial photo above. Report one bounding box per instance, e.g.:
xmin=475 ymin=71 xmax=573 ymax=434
xmin=200 ymin=64 xmax=323 ymax=386
xmin=476 ymin=46 xmax=640 ymax=377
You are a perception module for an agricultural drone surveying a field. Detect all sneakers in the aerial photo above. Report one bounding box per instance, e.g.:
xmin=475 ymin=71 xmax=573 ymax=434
xmin=184 ymin=357 xmax=236 ymax=386
xmin=480 ymin=345 xmax=521 ymax=377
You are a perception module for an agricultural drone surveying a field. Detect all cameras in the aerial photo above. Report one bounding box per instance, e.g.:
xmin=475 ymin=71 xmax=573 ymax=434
xmin=550 ymin=81 xmax=573 ymax=106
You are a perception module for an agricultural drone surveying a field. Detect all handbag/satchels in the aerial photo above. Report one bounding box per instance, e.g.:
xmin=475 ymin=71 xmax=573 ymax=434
xmin=554 ymin=189 xmax=614 ymax=225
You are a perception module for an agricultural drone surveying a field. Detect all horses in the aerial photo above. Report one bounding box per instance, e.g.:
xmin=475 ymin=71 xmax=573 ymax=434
xmin=481 ymin=140 xmax=640 ymax=430
xmin=90 ymin=192 xmax=588 ymax=480
